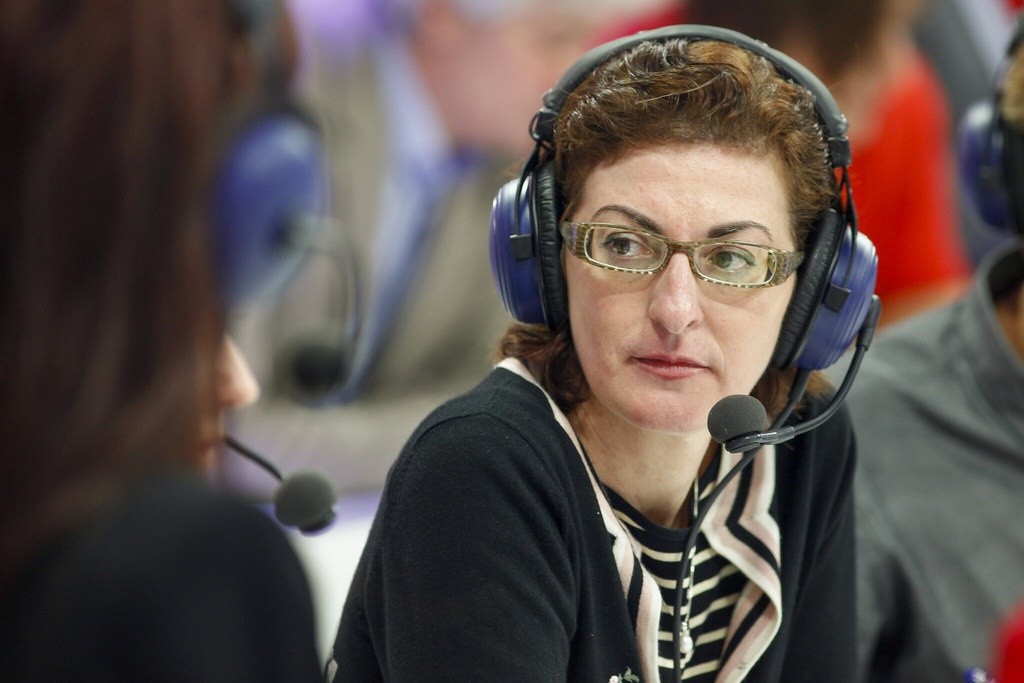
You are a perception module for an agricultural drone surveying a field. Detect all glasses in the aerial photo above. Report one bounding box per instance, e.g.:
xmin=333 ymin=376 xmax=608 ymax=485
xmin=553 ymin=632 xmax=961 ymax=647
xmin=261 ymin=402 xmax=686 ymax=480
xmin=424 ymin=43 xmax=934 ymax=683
xmin=558 ymin=218 xmax=808 ymax=289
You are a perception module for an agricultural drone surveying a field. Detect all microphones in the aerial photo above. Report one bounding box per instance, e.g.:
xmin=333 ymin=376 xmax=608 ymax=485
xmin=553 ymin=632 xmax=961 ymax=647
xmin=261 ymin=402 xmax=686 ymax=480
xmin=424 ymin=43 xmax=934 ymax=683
xmin=270 ymin=209 xmax=365 ymax=408
xmin=218 ymin=434 xmax=340 ymax=534
xmin=707 ymin=294 xmax=881 ymax=457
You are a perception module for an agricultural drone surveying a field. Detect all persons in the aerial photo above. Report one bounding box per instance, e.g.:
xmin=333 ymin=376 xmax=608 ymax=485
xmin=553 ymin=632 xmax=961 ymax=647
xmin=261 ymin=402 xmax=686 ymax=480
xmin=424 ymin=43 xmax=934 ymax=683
xmin=212 ymin=0 xmax=611 ymax=428
xmin=573 ymin=3 xmax=961 ymax=405
xmin=323 ymin=26 xmax=863 ymax=683
xmin=789 ymin=16 xmax=1022 ymax=683
xmin=1 ymin=1 xmax=320 ymax=683
xmin=162 ymin=0 xmax=347 ymax=340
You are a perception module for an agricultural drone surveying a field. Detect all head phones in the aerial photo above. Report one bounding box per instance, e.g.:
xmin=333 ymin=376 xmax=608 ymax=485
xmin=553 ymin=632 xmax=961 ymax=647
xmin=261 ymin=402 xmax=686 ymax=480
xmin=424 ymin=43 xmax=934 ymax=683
xmin=206 ymin=1 xmax=329 ymax=320
xmin=486 ymin=23 xmax=877 ymax=406
xmin=957 ymin=16 xmax=1024 ymax=234
